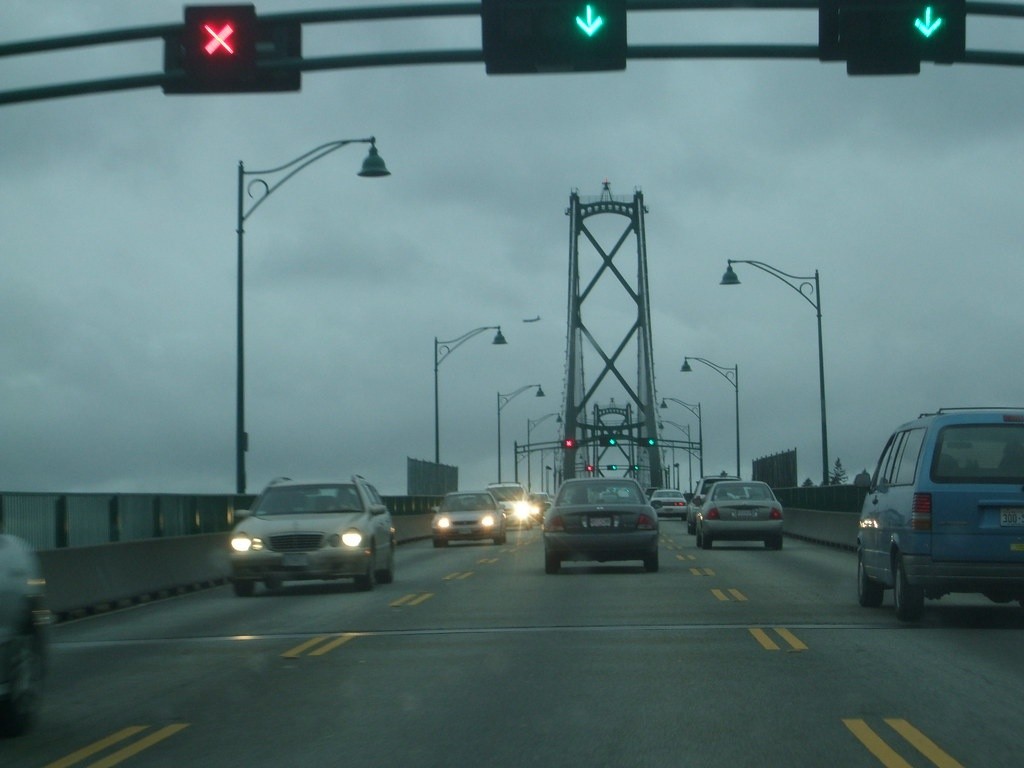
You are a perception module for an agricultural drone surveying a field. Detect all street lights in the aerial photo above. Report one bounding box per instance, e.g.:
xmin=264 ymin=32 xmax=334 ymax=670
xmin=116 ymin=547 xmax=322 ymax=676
xmin=236 ymin=131 xmax=391 ymax=496
xmin=527 ymin=413 xmax=563 ymax=491
xmin=658 ymin=419 xmax=692 ymax=495
xmin=660 ymin=396 xmax=704 ymax=477
xmin=436 ymin=324 xmax=509 ymax=465
xmin=679 ymin=356 xmax=740 ymax=477
xmin=720 ymin=257 xmax=830 ymax=487
xmin=498 ymin=383 xmax=547 ymax=484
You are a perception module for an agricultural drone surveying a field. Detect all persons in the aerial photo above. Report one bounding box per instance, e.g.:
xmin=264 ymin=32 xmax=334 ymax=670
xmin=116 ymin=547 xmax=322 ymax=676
xmin=718 ymin=488 xmax=727 ymax=498
xmin=327 ymin=488 xmax=359 ymax=510
xmin=473 ymin=495 xmax=487 ymax=509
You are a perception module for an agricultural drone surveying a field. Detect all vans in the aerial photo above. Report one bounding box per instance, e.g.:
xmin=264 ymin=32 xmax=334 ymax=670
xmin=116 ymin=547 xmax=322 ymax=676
xmin=853 ymin=405 xmax=1024 ymax=624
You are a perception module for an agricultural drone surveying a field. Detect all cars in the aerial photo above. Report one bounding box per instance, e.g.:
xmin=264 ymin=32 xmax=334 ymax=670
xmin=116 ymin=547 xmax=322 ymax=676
xmin=0 ymin=533 xmax=54 ymax=739
xmin=541 ymin=476 xmax=664 ymax=574
xmin=642 ymin=486 xmax=690 ymax=521
xmin=693 ymin=480 xmax=785 ymax=551
xmin=431 ymin=490 xmax=508 ymax=548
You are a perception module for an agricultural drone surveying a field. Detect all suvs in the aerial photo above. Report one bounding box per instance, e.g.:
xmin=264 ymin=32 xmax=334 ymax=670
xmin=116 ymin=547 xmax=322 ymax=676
xmin=481 ymin=482 xmax=554 ymax=531
xmin=226 ymin=475 xmax=396 ymax=598
xmin=684 ymin=475 xmax=747 ymax=535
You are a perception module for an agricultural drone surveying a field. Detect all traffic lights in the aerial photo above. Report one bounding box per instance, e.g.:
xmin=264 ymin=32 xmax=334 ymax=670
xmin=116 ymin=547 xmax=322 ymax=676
xmin=607 ymin=465 xmax=617 ymax=470
xmin=630 ymin=465 xmax=639 ymax=470
xmin=638 ymin=439 xmax=656 ymax=446
xmin=481 ymin=0 xmax=626 ymax=73
xmin=585 ymin=465 xmax=594 ymax=471
xmin=561 ymin=439 xmax=575 ymax=448
xmin=600 ymin=436 xmax=617 ymax=446
xmin=162 ymin=4 xmax=304 ymax=94
xmin=819 ymin=0 xmax=967 ymax=76
